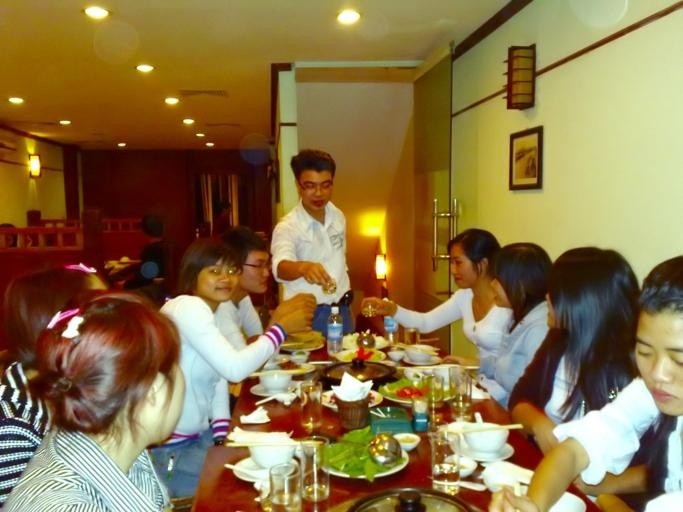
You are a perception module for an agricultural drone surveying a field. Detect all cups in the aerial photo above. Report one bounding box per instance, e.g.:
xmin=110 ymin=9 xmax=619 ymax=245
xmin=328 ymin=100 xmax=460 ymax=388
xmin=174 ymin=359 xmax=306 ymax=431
xmin=322 ymin=280 xmax=336 ymax=295
xmin=361 ymin=301 xmax=377 ymax=317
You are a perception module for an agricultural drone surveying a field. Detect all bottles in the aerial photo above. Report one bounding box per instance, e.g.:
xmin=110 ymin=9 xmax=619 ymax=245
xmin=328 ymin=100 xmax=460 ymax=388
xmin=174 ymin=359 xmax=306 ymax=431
xmin=326 ymin=306 xmax=343 ymax=357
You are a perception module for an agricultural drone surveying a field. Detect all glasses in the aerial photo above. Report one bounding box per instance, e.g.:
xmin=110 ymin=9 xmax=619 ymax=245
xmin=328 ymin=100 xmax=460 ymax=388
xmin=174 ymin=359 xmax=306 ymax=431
xmin=297 ymin=179 xmax=334 ymax=190
xmin=244 ymin=261 xmax=272 ymax=270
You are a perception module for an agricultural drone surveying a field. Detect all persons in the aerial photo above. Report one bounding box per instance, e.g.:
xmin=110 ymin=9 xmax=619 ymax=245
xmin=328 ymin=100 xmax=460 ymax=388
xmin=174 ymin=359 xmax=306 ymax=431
xmin=210 ymin=201 xmax=234 ymax=234
xmin=269 ymin=147 xmax=353 ymax=340
xmin=114 ymin=214 xmax=166 ymax=289
xmin=142 ymin=235 xmax=315 ymax=502
xmin=476 ymin=241 xmax=553 ymax=412
xmin=506 ymin=245 xmax=668 ymax=501
xmin=485 ymin=253 xmax=682 ymax=511
xmin=1 ymin=286 xmax=188 ymax=512
xmin=0 ymin=266 xmax=109 ymax=512
xmin=358 ymin=227 xmax=514 ymax=386
xmin=212 ymin=224 xmax=318 ymax=400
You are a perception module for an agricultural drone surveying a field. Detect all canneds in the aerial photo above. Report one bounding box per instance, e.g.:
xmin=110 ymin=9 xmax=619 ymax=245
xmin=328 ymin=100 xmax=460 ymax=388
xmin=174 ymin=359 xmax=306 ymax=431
xmin=403 ymin=328 xmax=418 ymax=346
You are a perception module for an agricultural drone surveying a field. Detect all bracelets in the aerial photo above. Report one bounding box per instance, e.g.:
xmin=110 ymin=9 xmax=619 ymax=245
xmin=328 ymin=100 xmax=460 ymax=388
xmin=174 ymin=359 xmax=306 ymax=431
xmin=214 ymin=440 xmax=222 ymax=445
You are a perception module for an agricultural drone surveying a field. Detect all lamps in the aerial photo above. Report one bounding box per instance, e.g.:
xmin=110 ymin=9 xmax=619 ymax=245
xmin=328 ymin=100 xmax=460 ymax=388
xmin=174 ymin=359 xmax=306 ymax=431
xmin=500 ymin=39 xmax=536 ymax=111
xmin=26 ymin=150 xmax=42 ymax=180
xmin=508 ymin=125 xmax=543 ymax=189
xmin=373 ymin=249 xmax=387 ymax=282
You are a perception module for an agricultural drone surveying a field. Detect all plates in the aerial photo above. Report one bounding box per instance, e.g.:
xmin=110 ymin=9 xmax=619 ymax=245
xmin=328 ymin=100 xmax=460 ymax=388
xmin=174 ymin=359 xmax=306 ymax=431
xmin=224 ymin=329 xmax=587 ymax=511
